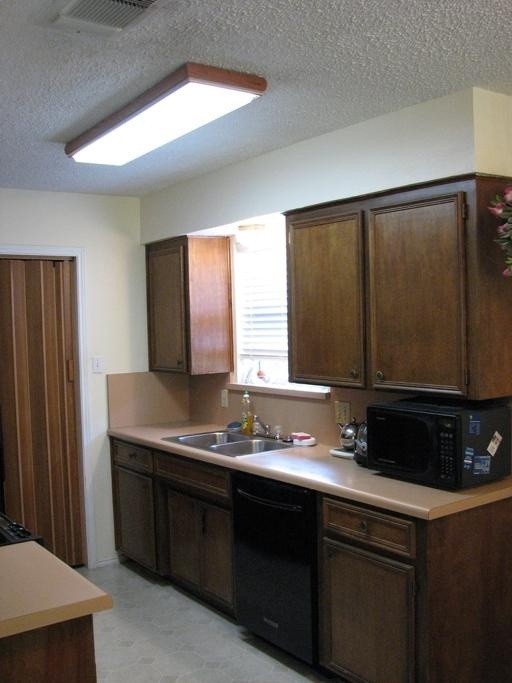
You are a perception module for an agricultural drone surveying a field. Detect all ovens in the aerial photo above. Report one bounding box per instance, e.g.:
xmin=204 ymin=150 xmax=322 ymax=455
xmin=230 ymin=471 xmax=318 ymax=667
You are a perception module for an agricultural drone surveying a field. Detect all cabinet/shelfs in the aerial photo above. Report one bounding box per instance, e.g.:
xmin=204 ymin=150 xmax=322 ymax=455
xmin=153 ymin=453 xmax=235 ymax=612
xmin=281 ymin=174 xmax=512 ymax=400
xmin=110 ymin=439 xmax=169 ymax=578
xmin=319 ymin=495 xmax=512 ymax=683
xmin=145 ymin=235 xmax=235 ymax=377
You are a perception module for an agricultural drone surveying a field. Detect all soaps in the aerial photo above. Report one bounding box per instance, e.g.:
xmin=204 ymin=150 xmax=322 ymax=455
xmin=290 ymin=431 xmax=312 ymax=441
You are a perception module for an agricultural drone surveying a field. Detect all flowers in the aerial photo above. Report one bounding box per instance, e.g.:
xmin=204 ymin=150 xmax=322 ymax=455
xmin=487 ymin=185 xmax=511 ymax=279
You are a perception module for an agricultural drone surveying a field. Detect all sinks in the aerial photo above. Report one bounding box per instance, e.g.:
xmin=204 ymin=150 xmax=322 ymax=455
xmin=210 ymin=440 xmax=295 ymax=458
xmin=161 ymin=430 xmax=249 ymax=448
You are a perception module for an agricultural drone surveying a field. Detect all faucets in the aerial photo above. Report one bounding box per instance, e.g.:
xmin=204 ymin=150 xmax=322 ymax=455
xmin=253 ymin=415 xmax=270 ymax=435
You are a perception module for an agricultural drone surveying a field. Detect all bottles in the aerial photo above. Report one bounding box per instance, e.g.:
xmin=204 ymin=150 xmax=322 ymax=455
xmin=239 ymin=394 xmax=254 ymax=435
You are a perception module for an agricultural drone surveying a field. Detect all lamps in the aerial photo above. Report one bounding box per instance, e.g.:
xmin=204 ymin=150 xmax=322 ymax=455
xmin=64 ymin=59 xmax=268 ymax=169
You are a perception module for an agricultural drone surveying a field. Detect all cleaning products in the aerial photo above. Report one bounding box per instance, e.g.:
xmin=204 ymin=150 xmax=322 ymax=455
xmin=239 ymin=390 xmax=253 ymax=434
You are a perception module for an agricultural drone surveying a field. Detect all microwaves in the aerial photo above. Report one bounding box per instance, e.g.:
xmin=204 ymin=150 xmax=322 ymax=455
xmin=367 ymin=397 xmax=511 ymax=489
